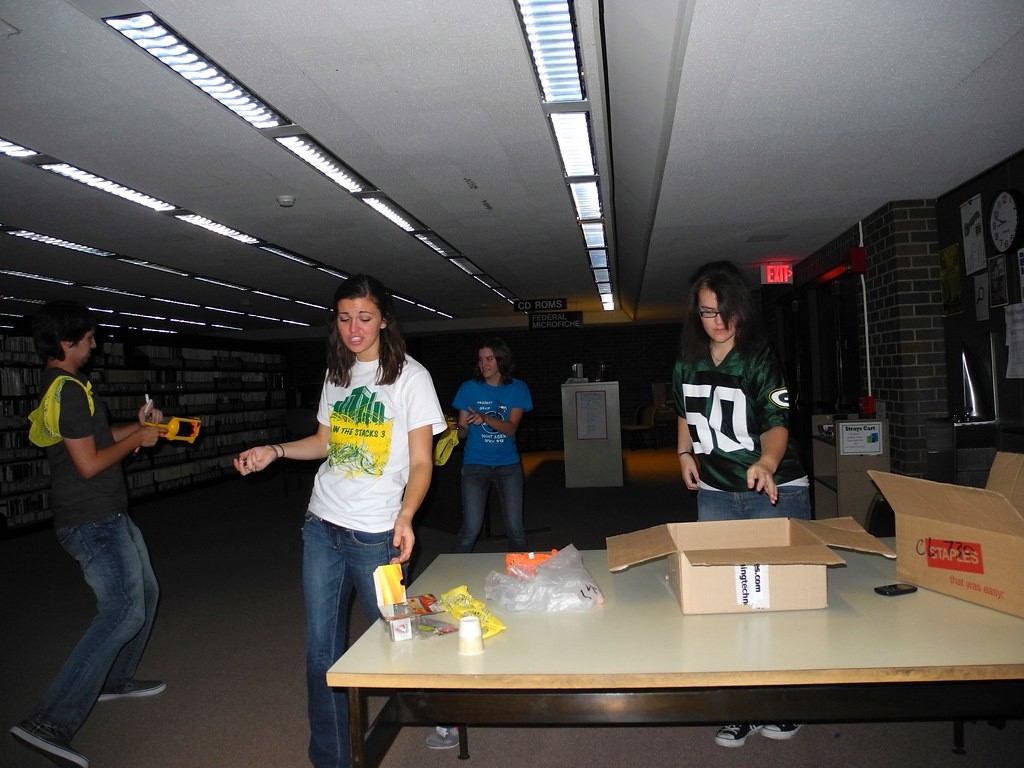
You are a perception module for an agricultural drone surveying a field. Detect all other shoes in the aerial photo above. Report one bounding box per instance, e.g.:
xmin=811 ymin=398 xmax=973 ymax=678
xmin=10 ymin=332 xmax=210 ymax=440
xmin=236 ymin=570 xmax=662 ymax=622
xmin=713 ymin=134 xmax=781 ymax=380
xmin=8 ymin=716 xmax=90 ymax=768
xmin=98 ymin=678 xmax=167 ymax=701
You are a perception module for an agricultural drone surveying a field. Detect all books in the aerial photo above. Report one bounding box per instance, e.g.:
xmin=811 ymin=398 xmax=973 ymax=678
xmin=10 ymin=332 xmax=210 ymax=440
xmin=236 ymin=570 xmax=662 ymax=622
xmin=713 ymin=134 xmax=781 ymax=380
xmin=0 ymin=336 xmax=291 ymax=527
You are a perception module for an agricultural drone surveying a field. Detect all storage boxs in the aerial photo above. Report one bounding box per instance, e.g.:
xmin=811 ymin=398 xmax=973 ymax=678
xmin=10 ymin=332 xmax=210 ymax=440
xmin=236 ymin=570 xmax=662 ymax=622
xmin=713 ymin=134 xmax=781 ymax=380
xmin=606 ymin=517 xmax=897 ymax=616
xmin=867 ymin=453 xmax=1024 ymax=618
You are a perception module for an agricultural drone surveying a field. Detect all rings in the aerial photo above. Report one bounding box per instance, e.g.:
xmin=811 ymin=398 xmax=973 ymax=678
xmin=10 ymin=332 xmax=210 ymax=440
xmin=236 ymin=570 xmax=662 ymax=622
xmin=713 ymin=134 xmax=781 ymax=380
xmin=252 ymin=469 xmax=256 ymax=472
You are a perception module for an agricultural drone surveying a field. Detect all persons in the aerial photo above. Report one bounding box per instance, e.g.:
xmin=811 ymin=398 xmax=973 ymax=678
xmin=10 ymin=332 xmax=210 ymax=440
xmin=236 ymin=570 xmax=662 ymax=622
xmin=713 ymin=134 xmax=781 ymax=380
xmin=231 ymin=274 xmax=462 ymax=768
xmin=673 ymin=261 xmax=810 ymax=746
xmin=10 ymin=301 xmax=168 ymax=768
xmin=451 ymin=338 xmax=533 ymax=552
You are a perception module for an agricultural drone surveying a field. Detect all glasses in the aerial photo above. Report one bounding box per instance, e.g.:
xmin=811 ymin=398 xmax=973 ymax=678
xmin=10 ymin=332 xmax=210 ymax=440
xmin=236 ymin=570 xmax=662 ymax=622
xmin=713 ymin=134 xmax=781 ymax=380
xmin=696 ymin=307 xmax=737 ymax=318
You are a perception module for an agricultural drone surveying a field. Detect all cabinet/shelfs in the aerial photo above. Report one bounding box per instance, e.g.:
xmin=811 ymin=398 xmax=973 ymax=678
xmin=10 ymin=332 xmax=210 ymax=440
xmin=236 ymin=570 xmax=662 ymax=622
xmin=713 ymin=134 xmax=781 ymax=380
xmin=0 ymin=331 xmax=302 ymax=535
xmin=812 ymin=413 xmax=891 ymax=532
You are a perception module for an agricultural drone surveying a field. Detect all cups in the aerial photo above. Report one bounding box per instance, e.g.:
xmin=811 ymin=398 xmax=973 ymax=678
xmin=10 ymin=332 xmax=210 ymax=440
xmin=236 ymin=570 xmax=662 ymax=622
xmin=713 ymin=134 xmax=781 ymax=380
xmin=458 ymin=617 xmax=486 ymax=656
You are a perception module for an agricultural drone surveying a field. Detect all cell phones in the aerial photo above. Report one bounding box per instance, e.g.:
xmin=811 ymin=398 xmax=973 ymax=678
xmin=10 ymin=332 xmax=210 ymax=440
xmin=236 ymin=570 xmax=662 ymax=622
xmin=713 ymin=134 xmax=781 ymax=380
xmin=874 ymin=584 xmax=917 ymax=597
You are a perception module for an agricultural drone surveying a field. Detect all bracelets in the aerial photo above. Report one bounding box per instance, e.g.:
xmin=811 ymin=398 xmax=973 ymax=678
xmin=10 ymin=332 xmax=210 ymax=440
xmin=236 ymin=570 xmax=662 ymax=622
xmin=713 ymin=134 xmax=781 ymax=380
xmin=270 ymin=445 xmax=278 ymax=459
xmin=278 ymin=444 xmax=284 ymax=457
xmin=678 ymin=452 xmax=691 ymax=458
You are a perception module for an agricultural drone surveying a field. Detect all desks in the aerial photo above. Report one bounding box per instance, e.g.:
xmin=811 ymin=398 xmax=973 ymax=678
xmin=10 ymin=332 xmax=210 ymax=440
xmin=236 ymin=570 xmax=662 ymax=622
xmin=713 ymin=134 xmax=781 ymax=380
xmin=326 ymin=535 xmax=1024 ymax=768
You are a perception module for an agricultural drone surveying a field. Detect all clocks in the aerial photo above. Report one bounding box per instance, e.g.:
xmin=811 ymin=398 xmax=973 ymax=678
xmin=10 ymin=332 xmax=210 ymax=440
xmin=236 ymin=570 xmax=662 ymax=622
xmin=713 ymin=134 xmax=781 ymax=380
xmin=988 ymin=187 xmax=1023 ymax=255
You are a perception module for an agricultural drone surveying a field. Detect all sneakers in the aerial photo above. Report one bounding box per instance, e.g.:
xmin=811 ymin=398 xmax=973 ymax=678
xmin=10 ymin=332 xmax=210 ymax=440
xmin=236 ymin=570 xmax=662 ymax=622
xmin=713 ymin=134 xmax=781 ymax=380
xmin=760 ymin=724 xmax=803 ymax=740
xmin=425 ymin=726 xmax=462 ymax=749
xmin=715 ymin=723 xmax=763 ymax=748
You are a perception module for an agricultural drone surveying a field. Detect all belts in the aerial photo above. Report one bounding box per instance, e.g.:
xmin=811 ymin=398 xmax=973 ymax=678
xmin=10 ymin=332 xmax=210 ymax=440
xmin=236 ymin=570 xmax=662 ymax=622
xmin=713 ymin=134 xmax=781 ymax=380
xmin=306 ymin=509 xmax=350 ymax=531
